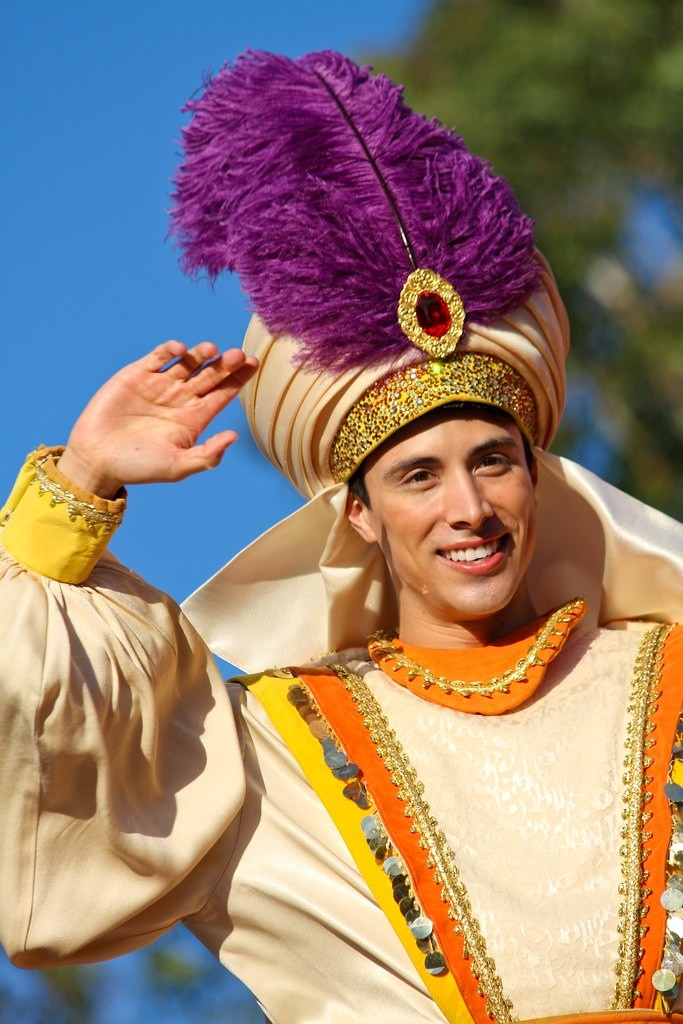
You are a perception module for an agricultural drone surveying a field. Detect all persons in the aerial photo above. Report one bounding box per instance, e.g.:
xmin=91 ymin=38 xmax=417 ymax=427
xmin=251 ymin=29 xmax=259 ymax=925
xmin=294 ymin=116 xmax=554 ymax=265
xmin=0 ymin=49 xmax=683 ymax=1024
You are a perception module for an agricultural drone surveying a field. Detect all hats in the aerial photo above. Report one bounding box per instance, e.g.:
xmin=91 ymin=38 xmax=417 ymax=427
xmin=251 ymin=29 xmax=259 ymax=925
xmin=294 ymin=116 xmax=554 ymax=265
xmin=172 ymin=50 xmax=678 ymax=675
